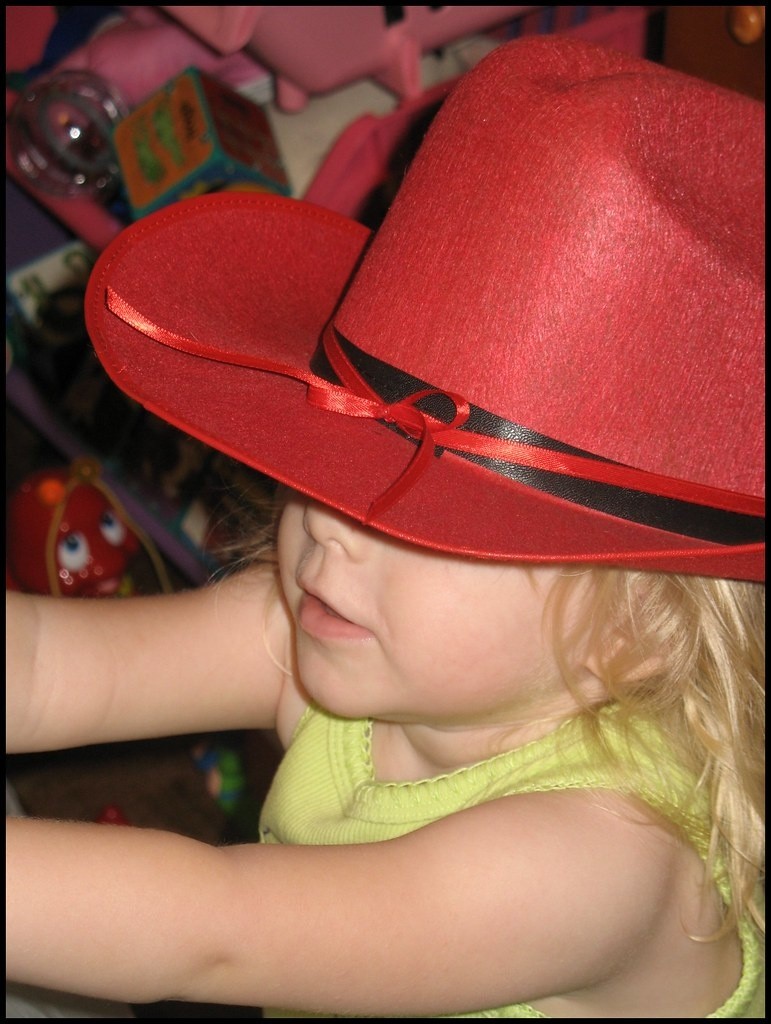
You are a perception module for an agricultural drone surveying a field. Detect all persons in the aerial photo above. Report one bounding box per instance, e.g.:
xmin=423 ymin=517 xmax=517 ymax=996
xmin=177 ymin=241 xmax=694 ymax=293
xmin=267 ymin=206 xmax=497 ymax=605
xmin=8 ymin=39 xmax=770 ymax=1016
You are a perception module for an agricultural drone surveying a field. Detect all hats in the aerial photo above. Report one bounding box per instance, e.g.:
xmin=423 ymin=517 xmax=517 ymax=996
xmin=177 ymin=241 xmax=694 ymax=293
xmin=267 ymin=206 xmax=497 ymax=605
xmin=84 ymin=29 xmax=764 ymax=585
